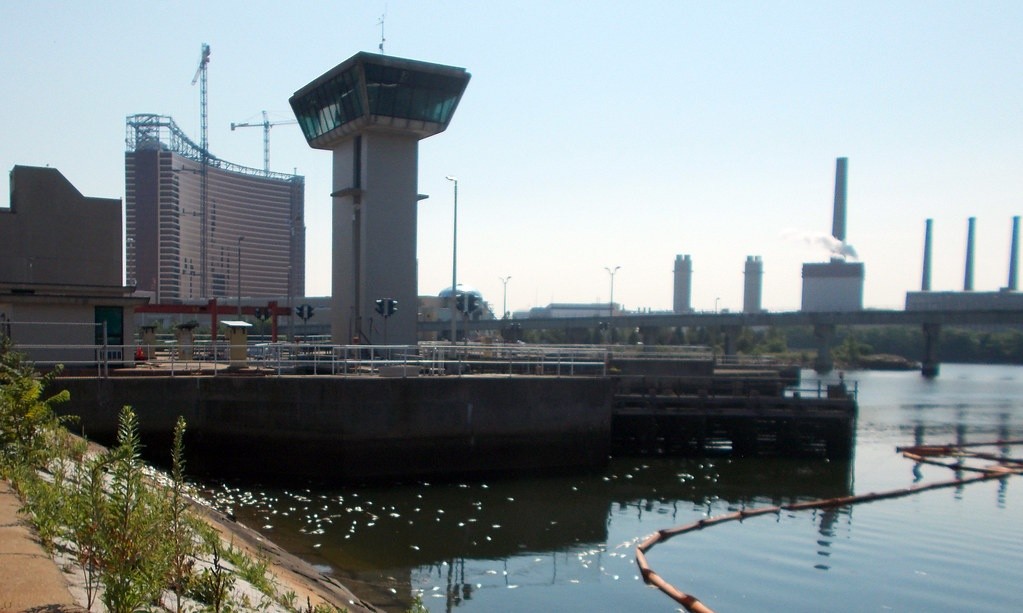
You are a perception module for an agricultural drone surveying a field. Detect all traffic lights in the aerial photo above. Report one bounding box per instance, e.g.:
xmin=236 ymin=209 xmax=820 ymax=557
xmin=265 ymin=306 xmax=272 ymax=320
xmin=469 ymin=294 xmax=480 ymax=313
xmin=296 ymin=305 xmax=304 ymax=318
xmin=387 ymin=299 xmax=398 ymax=316
xmin=376 ymin=299 xmax=384 ymax=314
xmin=307 ymin=304 xmax=315 ymax=319
xmin=255 ymin=307 xmax=261 ymax=319
xmin=456 ymin=293 xmax=465 ymax=312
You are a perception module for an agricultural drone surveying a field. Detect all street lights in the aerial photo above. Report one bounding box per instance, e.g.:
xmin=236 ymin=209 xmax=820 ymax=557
xmin=445 ymin=174 xmax=458 ymax=359
xmin=238 ymin=236 xmax=243 ymax=319
xmin=501 ymin=276 xmax=510 ymax=344
xmin=605 ymin=265 xmax=622 ymax=327
xmin=715 ymin=297 xmax=720 ymax=316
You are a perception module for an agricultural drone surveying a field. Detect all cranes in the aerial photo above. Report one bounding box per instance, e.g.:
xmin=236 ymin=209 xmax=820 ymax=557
xmin=232 ymin=109 xmax=301 ymax=174
xmin=190 ymin=40 xmax=213 ymax=297
